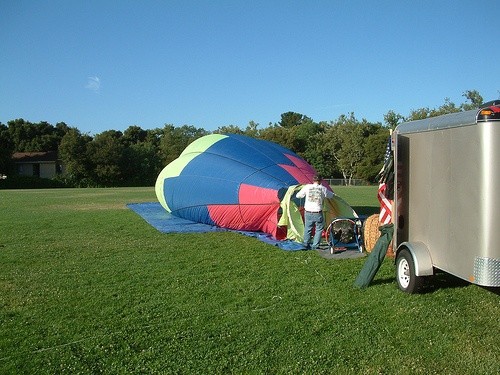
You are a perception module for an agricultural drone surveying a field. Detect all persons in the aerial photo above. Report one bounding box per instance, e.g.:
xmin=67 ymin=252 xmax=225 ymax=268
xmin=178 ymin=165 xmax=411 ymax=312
xmin=296 ymin=174 xmax=334 ymax=250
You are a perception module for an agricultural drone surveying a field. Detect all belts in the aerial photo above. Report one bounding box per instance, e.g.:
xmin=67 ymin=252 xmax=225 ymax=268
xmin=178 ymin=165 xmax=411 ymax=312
xmin=306 ymin=210 xmax=322 ymax=213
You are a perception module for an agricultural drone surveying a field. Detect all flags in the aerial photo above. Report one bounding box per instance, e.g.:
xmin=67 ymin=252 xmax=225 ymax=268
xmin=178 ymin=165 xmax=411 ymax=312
xmin=377 ymin=134 xmax=393 ymax=225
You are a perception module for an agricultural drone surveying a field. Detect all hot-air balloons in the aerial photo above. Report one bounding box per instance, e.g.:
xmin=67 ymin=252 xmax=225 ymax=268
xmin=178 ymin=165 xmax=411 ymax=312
xmin=152 ymin=131 xmax=395 ymax=257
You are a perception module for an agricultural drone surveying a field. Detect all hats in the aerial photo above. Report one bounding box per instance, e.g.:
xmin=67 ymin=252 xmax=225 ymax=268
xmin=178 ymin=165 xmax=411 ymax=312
xmin=313 ymin=174 xmax=322 ymax=181
